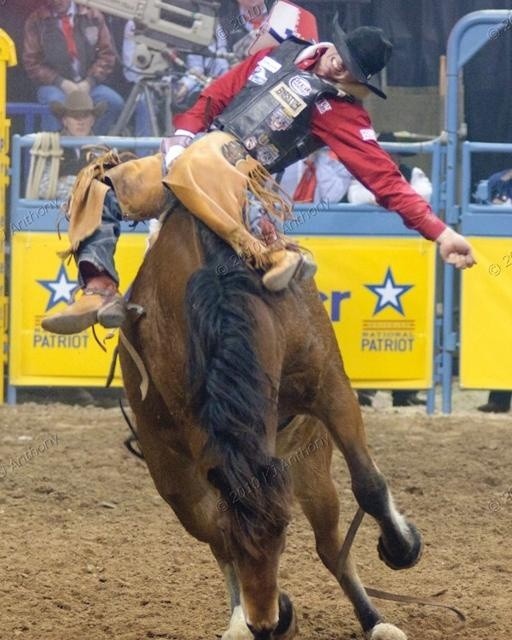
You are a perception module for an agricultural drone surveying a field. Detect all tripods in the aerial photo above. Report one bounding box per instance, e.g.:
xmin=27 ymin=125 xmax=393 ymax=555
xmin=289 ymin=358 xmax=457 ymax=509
xmin=107 ymin=78 xmax=176 ymax=137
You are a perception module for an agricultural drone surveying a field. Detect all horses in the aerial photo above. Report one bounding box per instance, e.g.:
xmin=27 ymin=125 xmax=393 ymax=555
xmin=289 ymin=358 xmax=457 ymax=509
xmin=113 ymin=197 xmax=424 ymax=640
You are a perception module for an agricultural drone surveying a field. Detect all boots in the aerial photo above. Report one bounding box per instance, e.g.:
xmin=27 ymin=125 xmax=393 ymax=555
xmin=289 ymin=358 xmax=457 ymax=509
xmin=40 ymin=288 xmax=126 ymax=336
xmin=262 ymin=240 xmax=319 ymax=293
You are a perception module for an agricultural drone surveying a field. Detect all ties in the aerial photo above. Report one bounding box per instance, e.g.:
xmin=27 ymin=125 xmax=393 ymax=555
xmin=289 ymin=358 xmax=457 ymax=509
xmin=60 ymin=13 xmax=79 ymax=63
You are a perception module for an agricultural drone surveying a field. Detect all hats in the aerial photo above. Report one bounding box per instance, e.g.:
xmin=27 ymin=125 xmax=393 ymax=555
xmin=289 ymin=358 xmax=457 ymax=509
xmin=331 ymin=10 xmax=394 ymax=100
xmin=49 ymin=89 xmax=108 ymax=120
